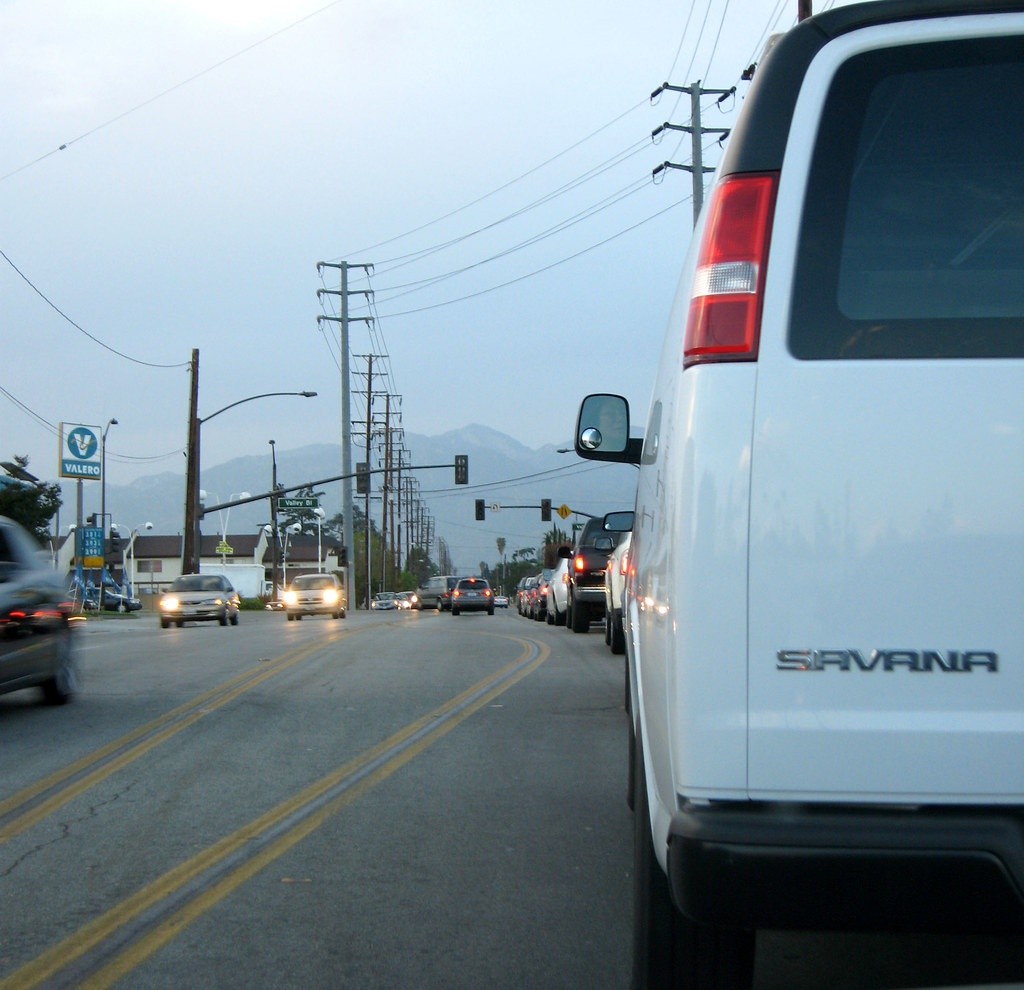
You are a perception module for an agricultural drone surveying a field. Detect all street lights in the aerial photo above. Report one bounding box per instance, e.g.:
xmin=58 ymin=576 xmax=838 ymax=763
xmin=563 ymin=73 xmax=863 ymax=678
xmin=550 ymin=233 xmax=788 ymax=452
xmin=200 ymin=489 xmax=251 ymax=577
xmin=268 ymin=439 xmax=279 ymax=602
xmin=100 ymin=417 xmax=120 ymax=538
xmin=190 ymin=391 xmax=317 ymax=573
xmin=111 ymin=521 xmax=154 ymax=597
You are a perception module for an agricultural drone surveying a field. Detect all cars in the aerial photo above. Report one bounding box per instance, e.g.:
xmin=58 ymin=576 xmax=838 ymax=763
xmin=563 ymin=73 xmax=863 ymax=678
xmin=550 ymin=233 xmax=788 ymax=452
xmin=371 ymin=591 xmax=419 ymax=610
xmin=63 ymin=587 xmax=144 ymax=612
xmin=515 ymin=514 xmax=633 ymax=655
xmin=448 ymin=577 xmax=497 ymax=616
xmin=158 ymin=572 xmax=240 ymax=628
xmin=0 ymin=512 xmax=79 ymax=705
xmin=494 ymin=595 xmax=509 ymax=609
xmin=63 ymin=594 xmax=100 ymax=615
xmin=283 ymin=573 xmax=347 ymax=621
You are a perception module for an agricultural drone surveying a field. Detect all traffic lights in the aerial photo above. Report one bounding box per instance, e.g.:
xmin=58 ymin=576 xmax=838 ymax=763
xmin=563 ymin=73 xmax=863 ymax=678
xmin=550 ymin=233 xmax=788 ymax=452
xmin=475 ymin=498 xmax=485 ymax=520
xmin=541 ymin=498 xmax=551 ymax=521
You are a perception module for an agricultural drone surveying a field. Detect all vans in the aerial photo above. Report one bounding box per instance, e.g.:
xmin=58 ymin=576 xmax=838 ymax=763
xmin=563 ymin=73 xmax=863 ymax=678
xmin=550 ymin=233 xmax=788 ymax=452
xmin=570 ymin=1 xmax=1024 ymax=990
xmin=412 ymin=575 xmax=460 ymax=612
